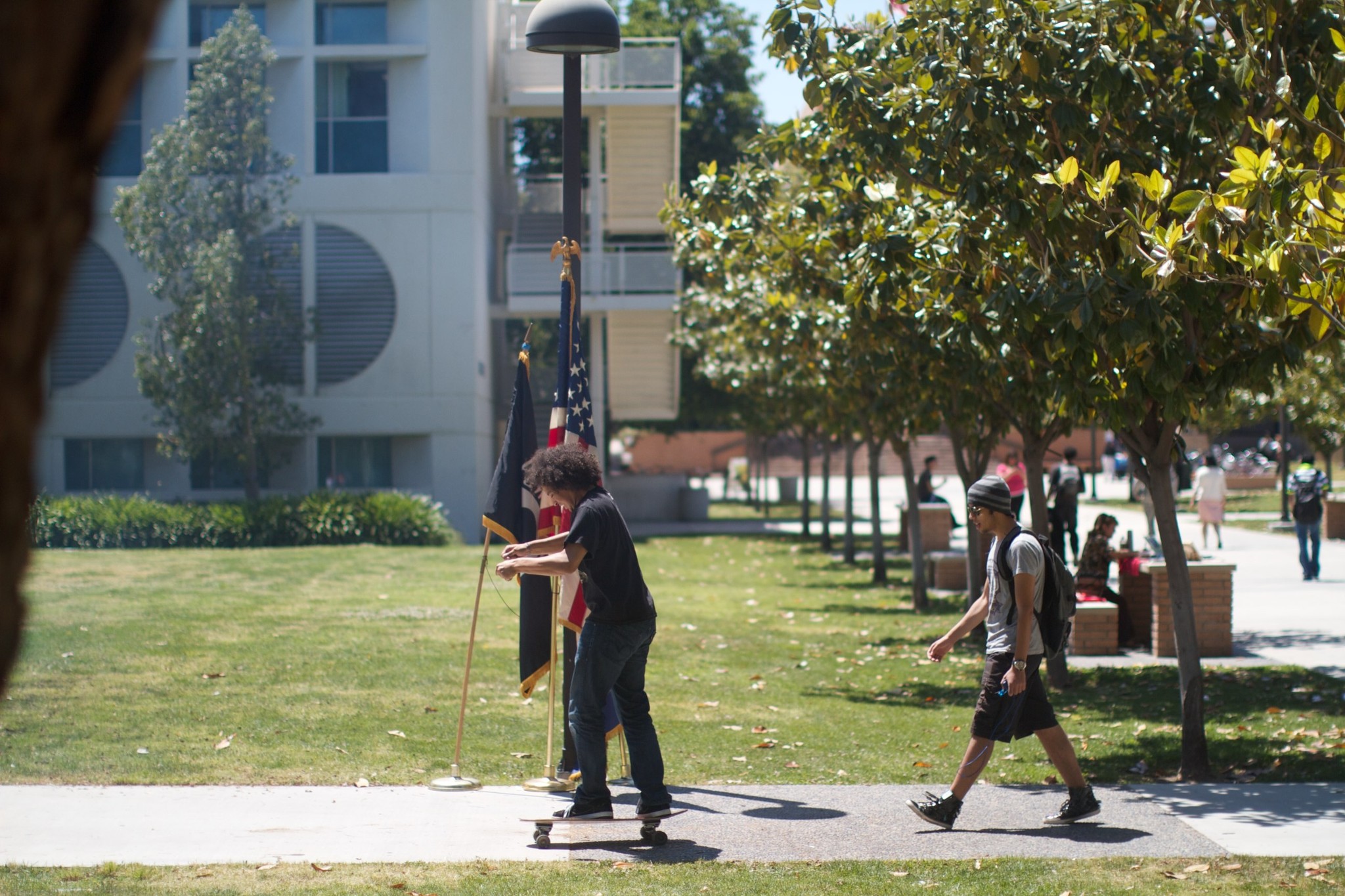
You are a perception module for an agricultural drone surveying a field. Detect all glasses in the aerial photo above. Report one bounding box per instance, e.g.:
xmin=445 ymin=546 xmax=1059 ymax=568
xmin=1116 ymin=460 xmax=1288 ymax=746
xmin=967 ymin=504 xmax=986 ymax=516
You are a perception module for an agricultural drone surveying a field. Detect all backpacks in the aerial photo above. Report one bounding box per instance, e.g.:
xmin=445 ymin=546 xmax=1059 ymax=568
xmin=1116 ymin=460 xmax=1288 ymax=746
xmin=996 ymin=525 xmax=1076 ymax=661
xmin=1290 ymin=470 xmax=1322 ymax=524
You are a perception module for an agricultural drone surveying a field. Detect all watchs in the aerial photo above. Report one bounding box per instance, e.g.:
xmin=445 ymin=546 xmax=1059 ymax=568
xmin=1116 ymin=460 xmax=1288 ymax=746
xmin=1012 ymin=659 xmax=1027 ymax=672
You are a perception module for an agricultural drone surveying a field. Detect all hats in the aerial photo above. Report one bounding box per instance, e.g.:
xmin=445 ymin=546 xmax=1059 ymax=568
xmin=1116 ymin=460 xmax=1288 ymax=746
xmin=967 ymin=475 xmax=1012 ymax=515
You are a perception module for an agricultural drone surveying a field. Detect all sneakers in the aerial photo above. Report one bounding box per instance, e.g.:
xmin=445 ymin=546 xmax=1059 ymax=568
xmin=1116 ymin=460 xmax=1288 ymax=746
xmin=552 ymin=801 xmax=616 ymax=819
xmin=634 ymin=796 xmax=672 ymax=818
xmin=1043 ymin=785 xmax=1101 ymax=823
xmin=906 ymin=791 xmax=963 ymax=829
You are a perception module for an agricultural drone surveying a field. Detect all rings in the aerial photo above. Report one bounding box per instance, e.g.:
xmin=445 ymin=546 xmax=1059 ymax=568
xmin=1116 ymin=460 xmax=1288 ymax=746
xmin=935 ymin=655 xmax=939 ymax=658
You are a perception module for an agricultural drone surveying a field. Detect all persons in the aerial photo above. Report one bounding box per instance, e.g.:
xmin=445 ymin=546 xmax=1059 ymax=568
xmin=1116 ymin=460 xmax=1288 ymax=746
xmin=1076 ymin=514 xmax=1151 ymax=647
xmin=1047 ymin=446 xmax=1085 ymax=565
xmin=905 ymin=475 xmax=1100 ymax=832
xmin=917 ymin=455 xmax=962 ymax=529
xmin=1287 ymin=452 xmax=1329 ymax=581
xmin=996 ymin=450 xmax=1027 ymax=521
xmin=1189 ymin=451 xmax=1226 ymax=549
xmin=1132 ymin=466 xmax=1156 ymax=536
xmin=1099 ymin=436 xmax=1128 ymax=483
xmin=494 ymin=445 xmax=673 ymax=819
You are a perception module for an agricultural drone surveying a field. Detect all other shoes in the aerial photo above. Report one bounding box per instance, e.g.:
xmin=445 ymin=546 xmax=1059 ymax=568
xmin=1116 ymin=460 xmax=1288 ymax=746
xmin=1303 ymin=567 xmax=1319 ymax=579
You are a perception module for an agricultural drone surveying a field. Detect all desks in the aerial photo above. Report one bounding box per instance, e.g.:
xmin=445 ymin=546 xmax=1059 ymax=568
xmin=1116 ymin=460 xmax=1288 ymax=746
xmin=896 ymin=503 xmax=950 ymax=553
xmin=1322 ymin=493 xmax=1345 ymax=539
xmin=1112 ymin=555 xmax=1236 ymax=657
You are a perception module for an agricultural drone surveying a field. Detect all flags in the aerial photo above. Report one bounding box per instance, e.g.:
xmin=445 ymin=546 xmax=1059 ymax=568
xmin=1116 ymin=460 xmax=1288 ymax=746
xmin=479 ymin=273 xmax=623 ymax=781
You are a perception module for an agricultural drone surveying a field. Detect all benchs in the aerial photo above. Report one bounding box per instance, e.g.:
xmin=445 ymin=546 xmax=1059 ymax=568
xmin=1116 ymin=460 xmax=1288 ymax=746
xmin=1067 ymin=601 xmax=1119 ymax=656
xmin=928 ymin=551 xmax=967 ymax=590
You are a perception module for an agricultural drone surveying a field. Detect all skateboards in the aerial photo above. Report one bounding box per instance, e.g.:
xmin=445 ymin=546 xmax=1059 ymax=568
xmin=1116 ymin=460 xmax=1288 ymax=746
xmin=517 ymin=808 xmax=689 ymax=849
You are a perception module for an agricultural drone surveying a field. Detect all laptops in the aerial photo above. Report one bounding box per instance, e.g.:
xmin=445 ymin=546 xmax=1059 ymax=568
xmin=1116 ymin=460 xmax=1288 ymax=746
xmin=1140 ymin=535 xmax=1165 ymax=558
